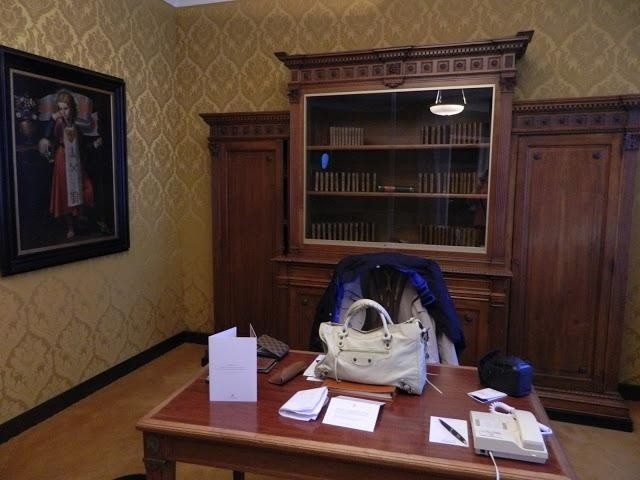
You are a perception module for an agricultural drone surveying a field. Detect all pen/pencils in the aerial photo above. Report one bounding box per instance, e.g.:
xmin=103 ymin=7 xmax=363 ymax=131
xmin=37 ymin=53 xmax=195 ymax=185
xmin=439 ymin=418 xmax=468 ymax=445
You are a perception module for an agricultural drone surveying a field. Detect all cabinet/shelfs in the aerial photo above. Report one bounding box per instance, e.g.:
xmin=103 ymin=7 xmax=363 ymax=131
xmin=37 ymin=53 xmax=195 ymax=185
xmin=197 ymin=110 xmax=291 ymax=364
xmin=273 ymin=29 xmax=538 ymax=369
xmin=508 ymin=95 xmax=639 ymax=434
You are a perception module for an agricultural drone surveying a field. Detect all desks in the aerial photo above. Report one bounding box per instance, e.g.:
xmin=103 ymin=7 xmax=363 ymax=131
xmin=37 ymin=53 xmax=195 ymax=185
xmin=135 ymin=349 xmax=579 ymax=480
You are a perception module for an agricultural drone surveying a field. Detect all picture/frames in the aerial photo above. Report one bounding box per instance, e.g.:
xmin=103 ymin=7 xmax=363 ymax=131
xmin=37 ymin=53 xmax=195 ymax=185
xmin=0 ymin=44 xmax=129 ymax=277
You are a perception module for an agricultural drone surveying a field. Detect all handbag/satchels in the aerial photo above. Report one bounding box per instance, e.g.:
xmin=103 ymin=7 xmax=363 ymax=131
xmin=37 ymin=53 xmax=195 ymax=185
xmin=314 ymin=297 xmax=428 ymax=395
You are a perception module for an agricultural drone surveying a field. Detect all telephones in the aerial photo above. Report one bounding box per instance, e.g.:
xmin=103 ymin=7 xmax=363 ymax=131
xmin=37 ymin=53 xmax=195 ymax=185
xmin=469 ymin=410 xmax=550 ymax=464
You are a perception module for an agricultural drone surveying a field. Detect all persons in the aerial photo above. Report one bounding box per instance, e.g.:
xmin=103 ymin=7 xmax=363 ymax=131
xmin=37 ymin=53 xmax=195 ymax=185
xmin=34 ymin=90 xmax=103 ymax=241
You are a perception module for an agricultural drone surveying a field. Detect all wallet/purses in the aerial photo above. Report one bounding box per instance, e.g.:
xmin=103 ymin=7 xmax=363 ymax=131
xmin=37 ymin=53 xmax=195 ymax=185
xmin=249 ymin=324 xmax=308 ymax=388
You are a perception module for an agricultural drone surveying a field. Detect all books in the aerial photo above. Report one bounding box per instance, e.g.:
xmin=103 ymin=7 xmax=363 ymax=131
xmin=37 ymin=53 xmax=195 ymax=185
xmin=415 ymin=120 xmax=484 ymax=248
xmin=467 ymin=387 xmax=508 ymax=403
xmin=307 ymin=119 xmax=379 ymax=242
xmin=322 ymin=378 xmax=399 ymax=404
xmin=255 ymin=355 xmax=278 ymax=373
xmin=378 ymin=184 xmax=415 ymax=193
xmin=256 ymin=334 xmax=289 ymax=358
